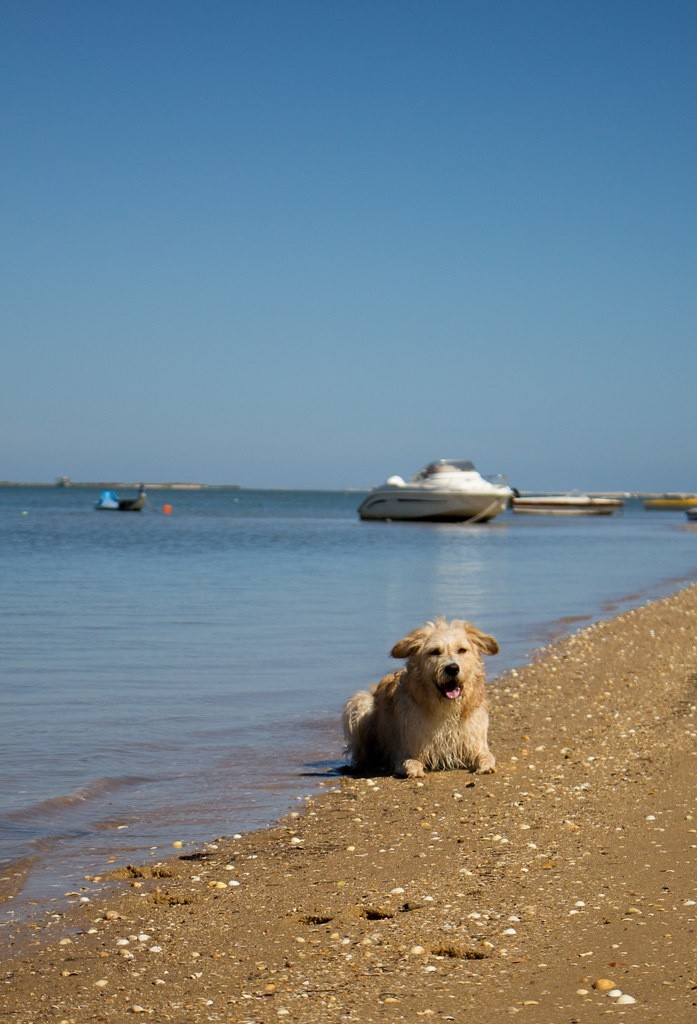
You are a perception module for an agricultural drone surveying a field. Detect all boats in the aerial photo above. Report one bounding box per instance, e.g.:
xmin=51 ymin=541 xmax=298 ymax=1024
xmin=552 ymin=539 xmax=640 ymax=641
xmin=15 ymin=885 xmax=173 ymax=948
xmin=94 ymin=484 xmax=145 ymax=511
xmin=514 ymin=495 xmax=626 ymax=517
xmin=643 ymin=498 xmax=697 ymax=509
xmin=356 ymin=456 xmax=514 ymax=525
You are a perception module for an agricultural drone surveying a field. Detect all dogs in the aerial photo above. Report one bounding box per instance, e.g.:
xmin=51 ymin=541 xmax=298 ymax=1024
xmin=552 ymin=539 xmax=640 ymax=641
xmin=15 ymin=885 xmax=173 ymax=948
xmin=340 ymin=613 xmax=500 ymax=780
